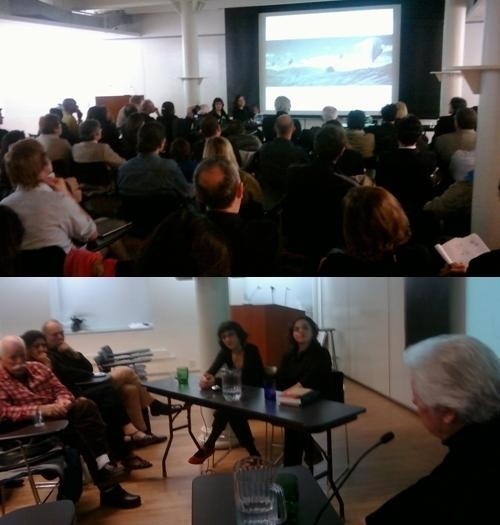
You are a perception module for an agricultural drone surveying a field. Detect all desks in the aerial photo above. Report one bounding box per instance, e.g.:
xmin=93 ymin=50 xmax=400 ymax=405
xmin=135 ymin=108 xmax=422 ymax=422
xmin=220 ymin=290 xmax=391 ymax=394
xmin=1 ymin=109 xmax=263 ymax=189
xmin=142 ymin=373 xmax=365 ymax=525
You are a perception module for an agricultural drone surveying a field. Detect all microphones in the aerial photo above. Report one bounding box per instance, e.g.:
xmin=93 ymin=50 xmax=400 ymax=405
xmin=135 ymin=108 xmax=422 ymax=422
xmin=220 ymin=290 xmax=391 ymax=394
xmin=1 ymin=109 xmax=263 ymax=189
xmin=311 ymin=429 xmax=395 ymax=524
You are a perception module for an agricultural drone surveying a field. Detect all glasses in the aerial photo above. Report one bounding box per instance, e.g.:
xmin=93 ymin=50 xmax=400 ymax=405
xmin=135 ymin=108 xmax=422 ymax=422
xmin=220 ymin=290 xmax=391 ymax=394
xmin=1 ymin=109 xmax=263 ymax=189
xmin=294 ymin=326 xmax=310 ymax=331
xmin=222 ymin=333 xmax=235 ymax=339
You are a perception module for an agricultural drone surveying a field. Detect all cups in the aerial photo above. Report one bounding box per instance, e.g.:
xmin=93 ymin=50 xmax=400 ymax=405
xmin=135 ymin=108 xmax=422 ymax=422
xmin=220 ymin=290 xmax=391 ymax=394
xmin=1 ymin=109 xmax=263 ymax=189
xmin=175 ymin=367 xmax=188 ymax=385
xmin=231 ymin=456 xmax=288 ymax=525
xmin=221 ymin=368 xmax=242 ymax=401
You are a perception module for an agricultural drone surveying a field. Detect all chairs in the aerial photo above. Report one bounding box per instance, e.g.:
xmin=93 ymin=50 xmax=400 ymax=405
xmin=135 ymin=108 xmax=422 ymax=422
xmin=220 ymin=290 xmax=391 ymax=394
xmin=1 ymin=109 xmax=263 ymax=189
xmin=200 ymin=365 xmax=278 ymax=473
xmin=0 ymin=348 xmax=156 ymax=525
xmin=269 ymin=369 xmax=349 ymax=492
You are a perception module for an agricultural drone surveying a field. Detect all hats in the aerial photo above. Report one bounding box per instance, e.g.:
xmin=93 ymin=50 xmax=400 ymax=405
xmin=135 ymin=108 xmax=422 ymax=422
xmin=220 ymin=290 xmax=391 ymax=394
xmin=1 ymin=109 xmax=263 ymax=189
xmin=137 ymin=100 xmax=156 ymax=114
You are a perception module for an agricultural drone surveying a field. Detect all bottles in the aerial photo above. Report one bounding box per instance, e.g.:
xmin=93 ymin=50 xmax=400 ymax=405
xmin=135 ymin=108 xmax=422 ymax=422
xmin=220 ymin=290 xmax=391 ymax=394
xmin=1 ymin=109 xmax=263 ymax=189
xmin=265 ymin=366 xmax=278 ymax=403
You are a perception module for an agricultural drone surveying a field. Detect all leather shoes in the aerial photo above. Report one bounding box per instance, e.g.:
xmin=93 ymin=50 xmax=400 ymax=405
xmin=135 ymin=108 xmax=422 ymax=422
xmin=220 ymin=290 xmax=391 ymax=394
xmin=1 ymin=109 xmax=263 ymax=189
xmin=142 ymin=429 xmax=167 ymax=445
xmin=108 ymin=468 xmax=127 ymax=482
xmin=99 ymin=491 xmax=142 ymax=510
xmin=149 ymin=400 xmax=182 ymax=416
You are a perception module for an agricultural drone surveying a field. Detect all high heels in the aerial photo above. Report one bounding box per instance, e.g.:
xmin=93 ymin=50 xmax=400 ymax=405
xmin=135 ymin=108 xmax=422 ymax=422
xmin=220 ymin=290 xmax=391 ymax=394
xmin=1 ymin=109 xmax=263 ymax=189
xmin=188 ymin=446 xmax=215 ymax=465
xmin=122 ymin=430 xmax=153 ymax=451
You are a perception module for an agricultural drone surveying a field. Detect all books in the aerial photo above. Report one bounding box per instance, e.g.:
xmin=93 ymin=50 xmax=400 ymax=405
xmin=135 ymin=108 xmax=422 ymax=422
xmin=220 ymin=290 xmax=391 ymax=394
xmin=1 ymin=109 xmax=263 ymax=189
xmin=279 ymin=383 xmax=320 ymax=405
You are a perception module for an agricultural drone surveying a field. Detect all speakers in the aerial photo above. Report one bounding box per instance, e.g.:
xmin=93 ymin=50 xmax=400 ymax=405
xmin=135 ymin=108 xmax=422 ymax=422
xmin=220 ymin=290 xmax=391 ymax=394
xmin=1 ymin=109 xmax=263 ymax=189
xmin=103 ymin=13 xmax=127 ymax=30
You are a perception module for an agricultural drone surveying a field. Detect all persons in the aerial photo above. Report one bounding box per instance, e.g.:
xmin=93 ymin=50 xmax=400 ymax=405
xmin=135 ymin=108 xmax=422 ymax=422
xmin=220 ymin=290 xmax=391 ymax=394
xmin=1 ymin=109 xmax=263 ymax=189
xmin=21 ymin=329 xmax=154 ymax=470
xmin=42 ymin=318 xmax=182 ymax=443
xmin=364 ymin=333 xmax=499 ymax=525
xmin=187 ymin=320 xmax=267 ymax=467
xmin=0 ymin=95 xmax=498 ymax=278
xmin=275 ymin=316 xmax=332 ymax=467
xmin=0 ymin=335 xmax=143 ymax=510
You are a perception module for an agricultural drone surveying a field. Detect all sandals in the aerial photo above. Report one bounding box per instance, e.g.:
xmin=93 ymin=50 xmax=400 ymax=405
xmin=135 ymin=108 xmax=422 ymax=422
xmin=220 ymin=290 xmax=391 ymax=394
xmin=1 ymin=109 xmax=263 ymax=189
xmin=122 ymin=454 xmax=153 ymax=469
